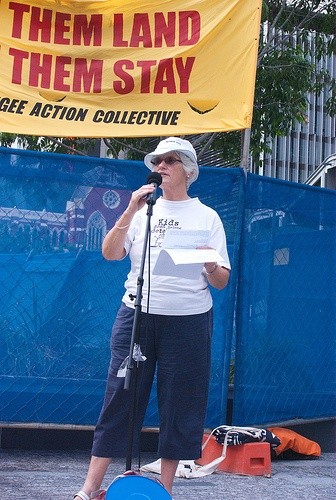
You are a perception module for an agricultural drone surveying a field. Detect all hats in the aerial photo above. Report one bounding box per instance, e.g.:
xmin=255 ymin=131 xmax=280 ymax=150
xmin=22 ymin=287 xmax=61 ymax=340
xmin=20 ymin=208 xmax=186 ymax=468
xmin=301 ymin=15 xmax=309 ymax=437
xmin=143 ymin=137 xmax=198 ymax=171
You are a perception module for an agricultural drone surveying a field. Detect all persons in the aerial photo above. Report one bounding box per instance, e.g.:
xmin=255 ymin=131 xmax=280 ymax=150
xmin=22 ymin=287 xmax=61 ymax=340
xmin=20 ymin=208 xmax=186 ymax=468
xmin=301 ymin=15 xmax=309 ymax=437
xmin=72 ymin=136 xmax=231 ymax=500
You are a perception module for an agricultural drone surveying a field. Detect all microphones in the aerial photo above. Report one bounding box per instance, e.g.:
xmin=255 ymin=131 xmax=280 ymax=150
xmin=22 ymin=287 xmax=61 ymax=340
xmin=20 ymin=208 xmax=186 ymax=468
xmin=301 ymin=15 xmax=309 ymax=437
xmin=147 ymin=172 xmax=162 ymax=205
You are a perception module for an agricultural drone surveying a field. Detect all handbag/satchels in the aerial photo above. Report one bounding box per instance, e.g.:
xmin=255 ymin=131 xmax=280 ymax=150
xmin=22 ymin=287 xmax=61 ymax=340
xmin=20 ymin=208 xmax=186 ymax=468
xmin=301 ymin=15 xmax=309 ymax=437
xmin=212 ymin=425 xmax=280 ymax=446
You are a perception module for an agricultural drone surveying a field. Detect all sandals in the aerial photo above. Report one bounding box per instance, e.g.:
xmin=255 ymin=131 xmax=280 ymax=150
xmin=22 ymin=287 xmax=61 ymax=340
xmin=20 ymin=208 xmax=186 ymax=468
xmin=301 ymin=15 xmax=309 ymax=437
xmin=74 ymin=489 xmax=105 ymax=500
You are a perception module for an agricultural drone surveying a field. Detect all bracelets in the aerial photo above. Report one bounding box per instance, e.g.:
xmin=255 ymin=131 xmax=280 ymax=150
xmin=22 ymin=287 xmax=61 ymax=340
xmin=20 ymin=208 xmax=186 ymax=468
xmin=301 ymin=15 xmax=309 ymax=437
xmin=114 ymin=221 xmax=130 ymax=229
xmin=204 ymin=264 xmax=218 ymax=275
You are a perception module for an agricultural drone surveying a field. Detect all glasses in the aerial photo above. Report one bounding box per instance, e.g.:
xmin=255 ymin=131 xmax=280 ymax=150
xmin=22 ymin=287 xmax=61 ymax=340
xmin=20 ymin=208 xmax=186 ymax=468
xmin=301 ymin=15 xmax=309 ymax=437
xmin=150 ymin=156 xmax=182 ymax=166
